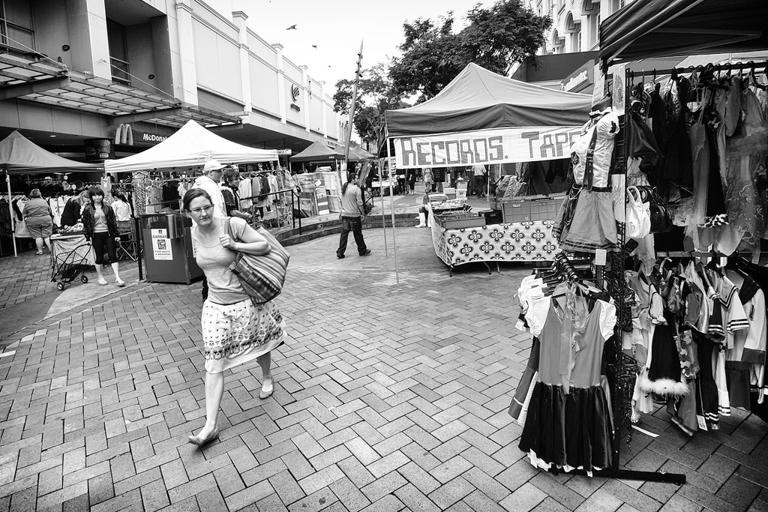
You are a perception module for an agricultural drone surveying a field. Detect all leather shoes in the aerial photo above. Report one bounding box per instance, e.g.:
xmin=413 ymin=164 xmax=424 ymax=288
xmin=259 ymin=376 xmax=274 ymax=399
xmin=188 ymin=427 xmax=219 ymax=445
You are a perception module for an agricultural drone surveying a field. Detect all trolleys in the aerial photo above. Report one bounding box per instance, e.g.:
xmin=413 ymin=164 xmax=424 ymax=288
xmin=50 ymin=244 xmax=92 ymax=291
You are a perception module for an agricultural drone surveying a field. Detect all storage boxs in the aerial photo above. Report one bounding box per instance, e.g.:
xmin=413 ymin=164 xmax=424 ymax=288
xmin=489 ymin=200 xmax=502 ymax=210
xmin=556 ymin=198 xmax=565 ymax=213
xmin=529 ymin=201 xmax=556 ymax=221
xmin=497 ymin=202 xmax=530 ymax=223
xmin=444 ymin=180 xmax=469 ymax=202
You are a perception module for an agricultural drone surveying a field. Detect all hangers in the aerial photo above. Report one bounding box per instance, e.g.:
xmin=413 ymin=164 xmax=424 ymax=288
xmin=1 ymin=168 xmax=288 ymax=202
xmin=629 ymin=249 xmax=766 ymax=279
xmin=533 ymin=250 xmax=588 ymax=295
xmin=625 ymin=60 xmax=767 ymax=90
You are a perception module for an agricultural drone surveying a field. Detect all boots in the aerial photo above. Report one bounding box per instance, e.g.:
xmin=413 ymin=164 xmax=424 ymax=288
xmin=414 ymin=212 xmax=426 ymax=227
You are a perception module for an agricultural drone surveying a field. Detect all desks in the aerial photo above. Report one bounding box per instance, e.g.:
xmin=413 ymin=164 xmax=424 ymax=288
xmin=49 ymin=231 xmax=136 ymax=273
xmin=424 ymin=200 xmax=579 ymax=274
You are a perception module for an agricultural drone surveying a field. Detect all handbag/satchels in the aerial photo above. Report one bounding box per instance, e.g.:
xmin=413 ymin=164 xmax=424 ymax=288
xmin=628 ymin=109 xmax=658 ymax=157
xmin=224 ymin=217 xmax=290 ymax=306
xmin=625 ymin=186 xmax=695 ymax=239
xmin=663 ymin=138 xmax=692 ymax=175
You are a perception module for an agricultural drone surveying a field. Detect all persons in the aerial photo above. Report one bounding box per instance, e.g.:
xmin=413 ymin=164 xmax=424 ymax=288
xmin=408 ymin=173 xmax=415 ymax=195
xmin=336 ymin=173 xmax=372 ymax=259
xmin=81 ymin=188 xmax=126 ymax=287
xmin=59 ymin=190 xmax=90 ymax=227
xmin=188 ymin=160 xmax=227 ymax=300
xmin=218 ymin=168 xmax=253 ymax=221
xmin=182 ymin=189 xmax=287 ymax=446
xmin=111 ymin=189 xmax=132 ymax=221
xmin=21 ymin=189 xmax=54 ymax=254
xmin=569 ymin=94 xmax=621 ymax=189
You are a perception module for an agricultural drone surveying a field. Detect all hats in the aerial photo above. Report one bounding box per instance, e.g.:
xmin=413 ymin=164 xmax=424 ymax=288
xmin=204 ymin=159 xmax=228 ymax=172
xmin=425 ymin=187 xmax=432 ymax=193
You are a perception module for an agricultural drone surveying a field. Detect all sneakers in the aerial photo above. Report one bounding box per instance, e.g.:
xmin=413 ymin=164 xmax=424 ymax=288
xmin=362 ymin=249 xmax=371 ymax=256
xmin=336 ymin=252 xmax=345 ymax=258
